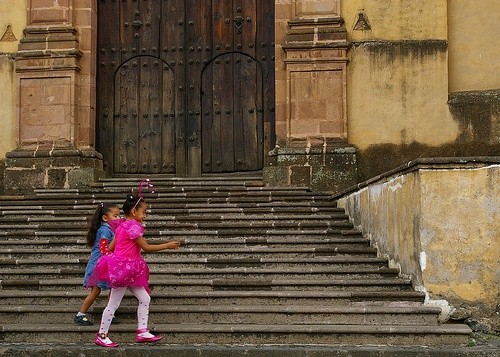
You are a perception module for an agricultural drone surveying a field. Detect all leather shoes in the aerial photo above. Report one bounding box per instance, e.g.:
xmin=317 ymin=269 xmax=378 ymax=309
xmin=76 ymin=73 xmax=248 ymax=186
xmin=136 ymin=327 xmax=163 ymax=342
xmin=95 ymin=332 xmax=119 ymax=347
xmin=111 ymin=316 xmax=120 ymax=324
xmin=74 ymin=315 xmax=93 ymax=326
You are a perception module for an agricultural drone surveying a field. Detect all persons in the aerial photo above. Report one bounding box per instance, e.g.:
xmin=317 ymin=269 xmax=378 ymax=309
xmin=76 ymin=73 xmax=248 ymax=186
xmin=95 ymin=194 xmax=181 ymax=347
xmin=72 ymin=202 xmax=120 ymax=325
